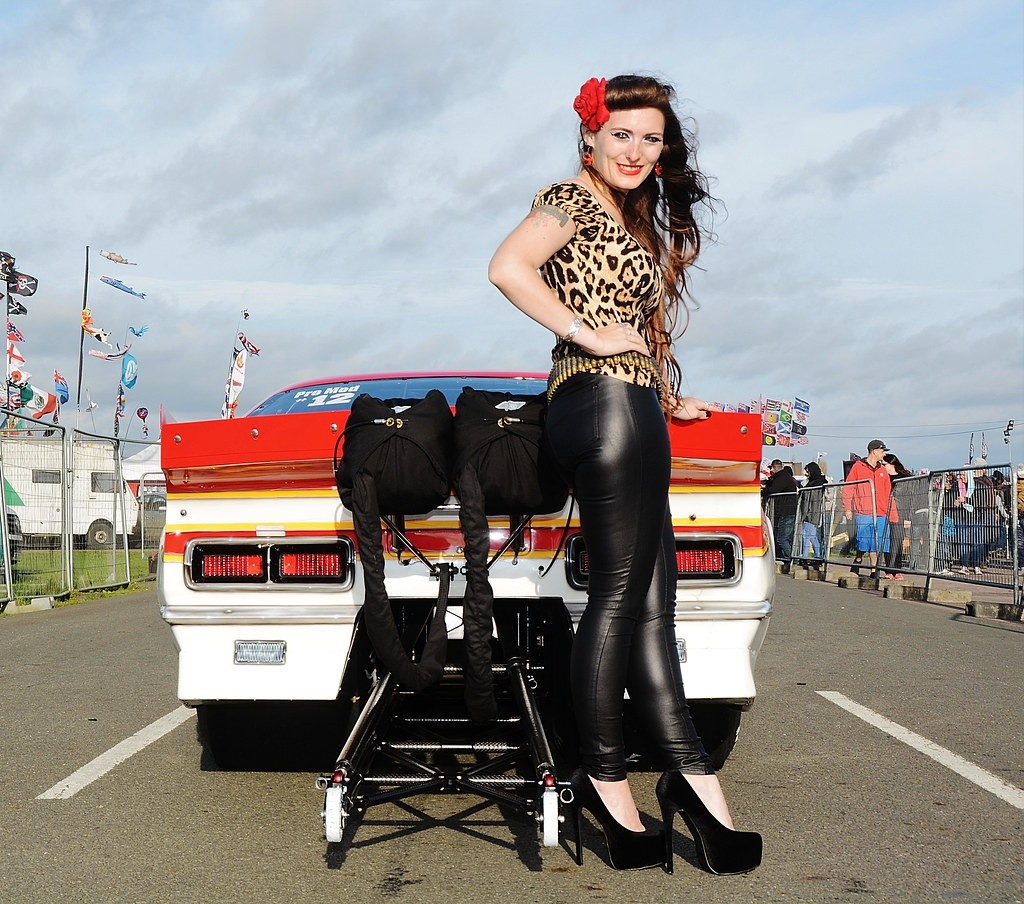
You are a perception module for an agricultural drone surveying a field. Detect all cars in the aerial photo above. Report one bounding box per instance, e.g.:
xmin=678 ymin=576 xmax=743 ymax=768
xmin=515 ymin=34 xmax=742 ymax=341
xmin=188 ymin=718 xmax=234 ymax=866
xmin=135 ymin=494 xmax=166 ymax=542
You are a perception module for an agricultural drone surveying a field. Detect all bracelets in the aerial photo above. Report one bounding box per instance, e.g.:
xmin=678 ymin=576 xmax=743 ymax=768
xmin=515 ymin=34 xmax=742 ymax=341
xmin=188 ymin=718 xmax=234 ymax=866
xmin=560 ymin=315 xmax=583 ymax=343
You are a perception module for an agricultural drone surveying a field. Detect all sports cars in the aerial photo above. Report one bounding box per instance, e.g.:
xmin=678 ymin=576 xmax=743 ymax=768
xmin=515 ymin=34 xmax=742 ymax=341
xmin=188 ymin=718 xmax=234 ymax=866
xmin=156 ymin=371 xmax=776 ymax=772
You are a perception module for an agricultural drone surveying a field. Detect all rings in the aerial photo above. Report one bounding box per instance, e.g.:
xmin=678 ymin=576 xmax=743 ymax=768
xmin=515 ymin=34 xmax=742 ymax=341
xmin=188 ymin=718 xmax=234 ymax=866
xmin=627 ymin=327 xmax=634 ymax=335
xmin=704 ymin=401 xmax=710 ymax=410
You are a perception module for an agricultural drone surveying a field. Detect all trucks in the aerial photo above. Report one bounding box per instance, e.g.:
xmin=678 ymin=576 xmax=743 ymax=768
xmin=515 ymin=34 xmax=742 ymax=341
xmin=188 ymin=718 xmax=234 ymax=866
xmin=1 ymin=438 xmax=139 ymax=550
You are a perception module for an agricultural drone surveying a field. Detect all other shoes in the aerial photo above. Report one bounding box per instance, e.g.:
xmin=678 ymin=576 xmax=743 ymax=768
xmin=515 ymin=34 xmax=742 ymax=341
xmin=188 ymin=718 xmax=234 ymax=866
xmin=933 ymin=569 xmax=954 ymax=576
xmin=850 ymin=557 xmax=862 ymax=574
xmin=958 ymin=567 xmax=983 ymax=575
xmin=884 ymin=573 xmax=904 ymax=581
xmin=869 ymin=572 xmax=876 ymax=579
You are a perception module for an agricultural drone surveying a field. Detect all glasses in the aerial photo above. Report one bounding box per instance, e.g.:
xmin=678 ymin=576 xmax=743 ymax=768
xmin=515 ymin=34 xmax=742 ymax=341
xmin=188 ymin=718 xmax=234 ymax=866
xmin=882 ymin=464 xmax=888 ymax=466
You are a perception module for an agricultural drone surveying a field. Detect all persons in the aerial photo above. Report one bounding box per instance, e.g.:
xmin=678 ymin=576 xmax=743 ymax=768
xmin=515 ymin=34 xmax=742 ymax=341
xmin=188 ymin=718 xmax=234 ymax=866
xmin=763 ymin=439 xmax=1024 ymax=579
xmin=487 ymin=75 xmax=763 ymax=876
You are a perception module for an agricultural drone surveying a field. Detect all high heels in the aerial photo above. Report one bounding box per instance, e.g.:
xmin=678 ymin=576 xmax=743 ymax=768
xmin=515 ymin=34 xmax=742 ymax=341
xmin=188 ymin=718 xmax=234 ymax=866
xmin=571 ymin=766 xmax=763 ymax=876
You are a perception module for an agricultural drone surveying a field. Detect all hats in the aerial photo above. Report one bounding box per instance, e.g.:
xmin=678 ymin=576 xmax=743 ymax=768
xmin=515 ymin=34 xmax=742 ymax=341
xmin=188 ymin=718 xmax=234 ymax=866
xmin=867 ymin=440 xmax=890 ymax=451
xmin=768 ymin=459 xmax=783 ymax=468
xmin=1017 ymin=463 xmax=1024 ymax=478
xmin=974 ymin=457 xmax=987 ymax=466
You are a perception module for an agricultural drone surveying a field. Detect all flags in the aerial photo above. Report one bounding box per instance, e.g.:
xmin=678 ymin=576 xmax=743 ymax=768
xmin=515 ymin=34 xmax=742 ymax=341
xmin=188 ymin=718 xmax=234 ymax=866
xmin=0 ymin=251 xmax=55 ymax=420
xmin=714 ymin=398 xmax=810 ymax=447
xmin=54 ymin=369 xmax=68 ymax=405
